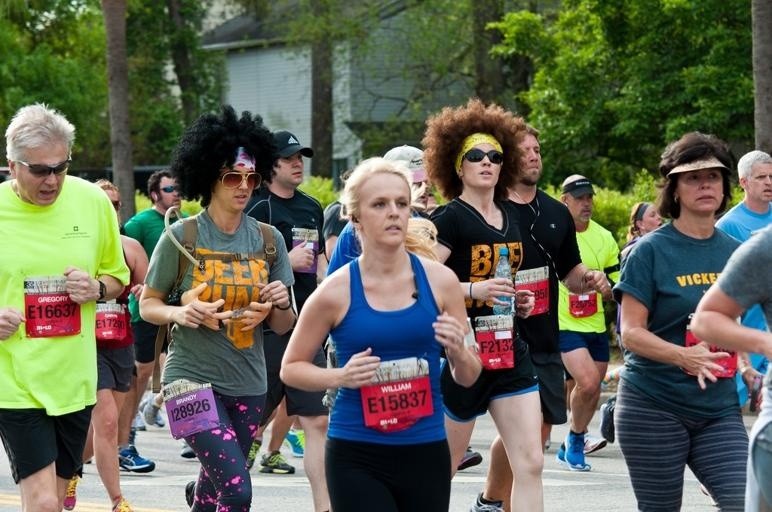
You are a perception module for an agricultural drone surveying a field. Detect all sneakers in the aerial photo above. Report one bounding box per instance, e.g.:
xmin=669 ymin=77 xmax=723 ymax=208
xmin=112 ymin=497 xmax=135 ymax=512
xmin=468 ymin=493 xmax=505 ymax=512
xmin=180 ymin=439 xmax=196 ymax=459
xmin=456 ymin=446 xmax=483 ymax=472
xmin=126 ymin=393 xmax=165 ymax=448
xmin=62 ymin=475 xmax=79 ymax=511
xmin=118 ymin=445 xmax=156 ymax=474
xmin=543 ymin=370 xmax=620 ymax=472
xmin=243 ymin=426 xmax=307 ymax=474
xmin=185 ymin=481 xmax=196 ymax=508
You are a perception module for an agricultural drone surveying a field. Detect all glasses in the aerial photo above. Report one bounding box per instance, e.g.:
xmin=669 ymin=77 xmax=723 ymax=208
xmin=216 ymin=170 xmax=263 ymax=189
xmin=110 ymin=200 xmax=122 ymax=211
xmin=9 ymin=154 xmax=73 ymax=178
xmin=463 ymin=149 xmax=506 ymax=165
xmin=158 ymin=185 xmax=181 ymax=193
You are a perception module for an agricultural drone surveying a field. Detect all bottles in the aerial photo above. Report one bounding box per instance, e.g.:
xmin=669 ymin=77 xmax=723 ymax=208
xmin=492 ymin=248 xmax=515 ymax=316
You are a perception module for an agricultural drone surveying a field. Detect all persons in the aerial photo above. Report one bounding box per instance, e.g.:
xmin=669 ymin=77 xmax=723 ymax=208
xmin=1 ymin=100 xmax=771 ymax=512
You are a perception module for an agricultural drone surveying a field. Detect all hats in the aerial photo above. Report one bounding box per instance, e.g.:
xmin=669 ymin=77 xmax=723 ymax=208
xmin=561 ymin=180 xmax=597 ymax=199
xmin=384 ymin=144 xmax=430 ymax=183
xmin=273 ymin=130 xmax=315 ymax=160
xmin=665 ymin=154 xmax=734 ymax=182
